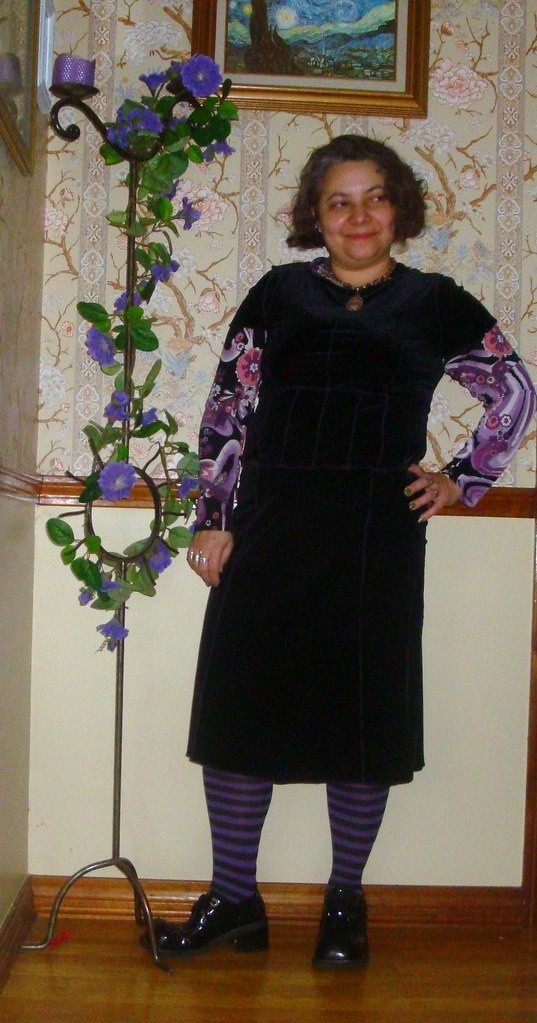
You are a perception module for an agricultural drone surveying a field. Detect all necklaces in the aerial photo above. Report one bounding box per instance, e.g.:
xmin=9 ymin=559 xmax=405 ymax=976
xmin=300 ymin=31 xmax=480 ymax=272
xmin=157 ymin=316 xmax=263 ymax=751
xmin=324 ymin=254 xmax=396 ymax=314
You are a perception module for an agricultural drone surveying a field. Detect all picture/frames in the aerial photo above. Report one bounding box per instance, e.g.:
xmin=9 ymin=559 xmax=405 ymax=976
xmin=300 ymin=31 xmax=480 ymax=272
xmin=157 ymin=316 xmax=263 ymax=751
xmin=0 ymin=0 xmax=42 ymax=177
xmin=188 ymin=1 xmax=433 ymax=118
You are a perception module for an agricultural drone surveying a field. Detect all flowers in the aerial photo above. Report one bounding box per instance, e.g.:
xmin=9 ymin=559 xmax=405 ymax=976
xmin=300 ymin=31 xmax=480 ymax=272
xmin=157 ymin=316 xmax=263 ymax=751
xmin=49 ymin=51 xmax=238 ymax=652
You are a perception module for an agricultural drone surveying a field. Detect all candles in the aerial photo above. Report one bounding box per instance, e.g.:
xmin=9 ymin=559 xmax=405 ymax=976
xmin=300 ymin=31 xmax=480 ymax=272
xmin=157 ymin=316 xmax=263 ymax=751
xmin=52 ymin=52 xmax=96 ymax=87
xmin=2 ymin=52 xmax=24 ymax=95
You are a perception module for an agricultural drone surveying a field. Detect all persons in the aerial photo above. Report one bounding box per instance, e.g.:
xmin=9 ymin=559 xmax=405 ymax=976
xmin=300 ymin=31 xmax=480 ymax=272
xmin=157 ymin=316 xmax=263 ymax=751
xmin=137 ymin=135 xmax=535 ymax=980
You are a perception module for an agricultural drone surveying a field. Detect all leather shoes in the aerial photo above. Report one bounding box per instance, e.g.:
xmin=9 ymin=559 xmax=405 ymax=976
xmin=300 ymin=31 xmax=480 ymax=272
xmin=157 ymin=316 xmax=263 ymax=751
xmin=312 ymin=886 xmax=369 ymax=970
xmin=141 ymin=893 xmax=268 ymax=956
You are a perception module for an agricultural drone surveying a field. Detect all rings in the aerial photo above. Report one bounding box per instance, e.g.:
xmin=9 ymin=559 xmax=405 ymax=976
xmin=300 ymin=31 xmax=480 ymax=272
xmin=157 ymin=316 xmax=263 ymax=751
xmin=430 ymin=487 xmax=438 ymax=498
xmin=425 ymin=476 xmax=435 ymax=489
xmin=200 ymin=557 xmax=210 ymax=563
xmin=187 ymin=550 xmax=194 ymax=559
xmin=194 ymin=553 xmax=201 ymax=562
